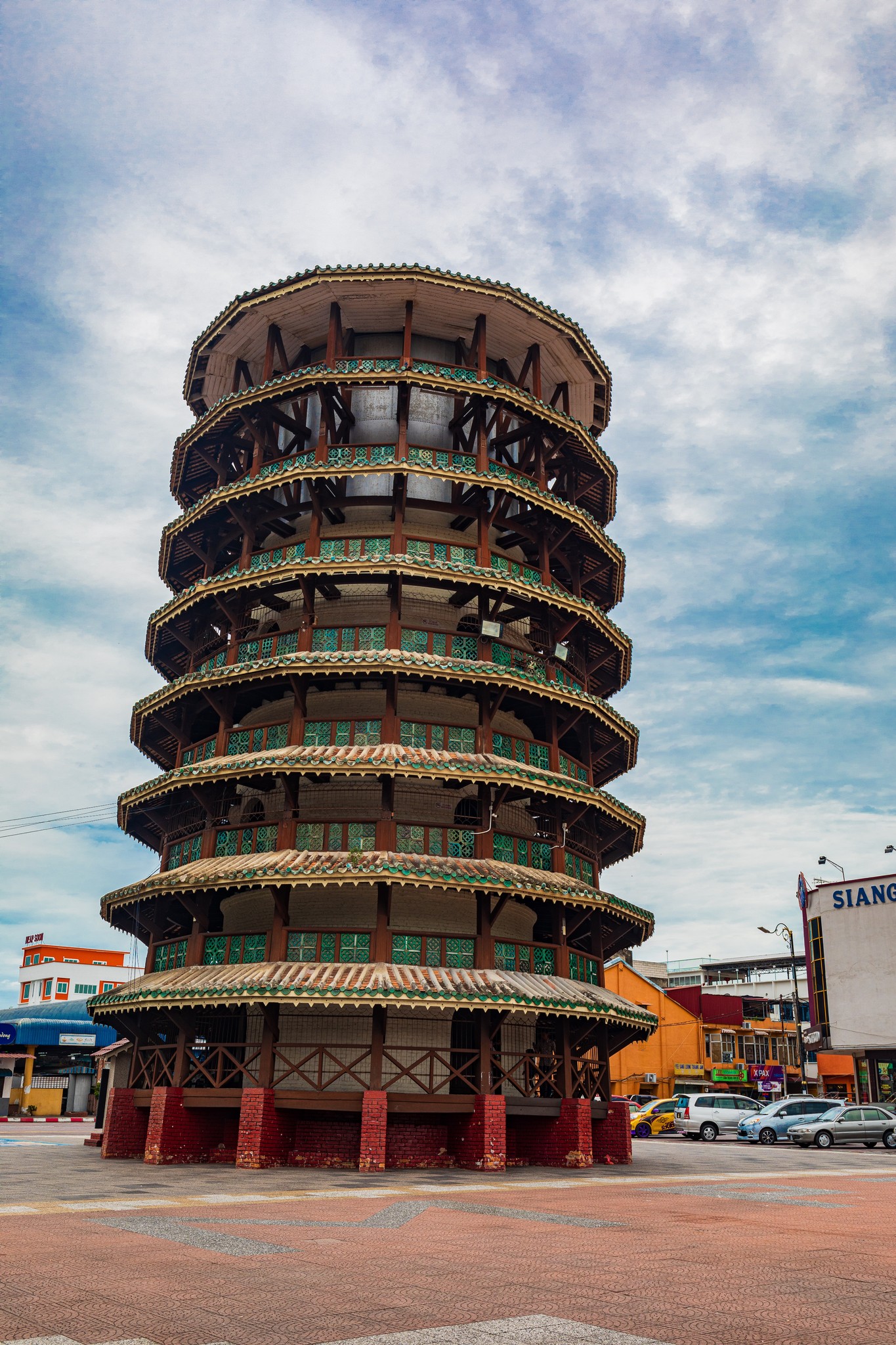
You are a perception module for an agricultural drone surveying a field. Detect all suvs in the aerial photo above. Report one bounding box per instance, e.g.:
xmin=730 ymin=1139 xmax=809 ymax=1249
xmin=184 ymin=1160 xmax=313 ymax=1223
xmin=737 ymin=1098 xmax=850 ymax=1146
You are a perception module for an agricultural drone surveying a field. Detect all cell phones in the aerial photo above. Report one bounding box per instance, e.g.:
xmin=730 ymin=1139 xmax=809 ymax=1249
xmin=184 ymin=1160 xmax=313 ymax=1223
xmin=532 ymin=1042 xmax=537 ymax=1049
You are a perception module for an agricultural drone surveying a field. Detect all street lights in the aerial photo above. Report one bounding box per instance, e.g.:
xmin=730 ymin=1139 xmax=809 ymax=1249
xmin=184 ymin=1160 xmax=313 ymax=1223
xmin=757 ymin=923 xmax=807 ymax=1094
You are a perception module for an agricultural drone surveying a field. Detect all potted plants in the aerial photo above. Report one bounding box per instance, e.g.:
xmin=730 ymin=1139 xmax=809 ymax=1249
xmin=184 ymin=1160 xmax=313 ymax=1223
xmin=28 ymin=1105 xmax=37 ymax=1116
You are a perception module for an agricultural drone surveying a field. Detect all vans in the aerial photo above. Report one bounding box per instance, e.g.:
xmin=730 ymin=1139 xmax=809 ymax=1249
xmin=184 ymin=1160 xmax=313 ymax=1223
xmin=672 ymin=1093 xmax=767 ymax=1142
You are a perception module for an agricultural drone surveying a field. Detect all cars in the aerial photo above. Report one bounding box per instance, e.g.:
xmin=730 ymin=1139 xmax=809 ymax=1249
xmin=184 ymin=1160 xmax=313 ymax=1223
xmin=840 ymin=1099 xmax=896 ymax=1115
xmin=786 ymin=1105 xmax=896 ymax=1149
xmin=580 ymin=1091 xmax=817 ymax=1139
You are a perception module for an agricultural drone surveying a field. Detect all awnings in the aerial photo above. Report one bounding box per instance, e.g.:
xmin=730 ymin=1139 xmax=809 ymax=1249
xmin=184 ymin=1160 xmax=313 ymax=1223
xmin=720 ymin=1028 xmax=736 ymax=1034
xmin=754 ymin=1031 xmax=769 ymax=1036
xmin=787 ymin=1033 xmax=796 ymax=1037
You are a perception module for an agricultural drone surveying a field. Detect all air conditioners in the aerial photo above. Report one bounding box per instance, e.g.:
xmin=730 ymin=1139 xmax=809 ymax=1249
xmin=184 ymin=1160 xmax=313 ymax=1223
xmin=743 ymin=1022 xmax=751 ymax=1029
xmin=736 ymin=1063 xmax=744 ymax=1070
xmin=645 ymin=1073 xmax=656 ymax=1082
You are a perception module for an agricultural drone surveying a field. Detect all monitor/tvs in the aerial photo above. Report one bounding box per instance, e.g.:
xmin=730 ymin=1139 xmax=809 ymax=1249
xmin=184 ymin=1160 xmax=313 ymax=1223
xmin=553 ymin=642 xmax=570 ymax=662
xmin=482 ymin=620 xmax=501 ymax=638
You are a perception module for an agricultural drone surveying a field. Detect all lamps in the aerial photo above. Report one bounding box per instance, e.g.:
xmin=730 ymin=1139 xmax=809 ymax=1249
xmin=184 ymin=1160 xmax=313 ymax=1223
xmin=480 ymin=619 xmax=503 ymax=639
xmin=885 ymin=845 xmax=896 ymax=854
xmin=548 ymin=642 xmax=570 ymax=664
xmin=817 ymin=856 xmax=845 ymax=883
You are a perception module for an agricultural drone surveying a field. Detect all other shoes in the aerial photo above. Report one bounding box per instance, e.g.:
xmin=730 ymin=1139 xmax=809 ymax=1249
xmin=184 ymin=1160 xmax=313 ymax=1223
xmin=534 ymin=1095 xmax=540 ymax=1098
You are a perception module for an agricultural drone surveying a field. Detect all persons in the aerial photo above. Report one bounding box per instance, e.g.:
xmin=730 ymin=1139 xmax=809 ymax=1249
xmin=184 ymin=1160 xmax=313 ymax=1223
xmin=522 ymin=1048 xmax=540 ymax=1098
xmin=533 ymin=1032 xmax=556 ymax=1097
xmin=475 ymin=1041 xmax=500 ymax=1088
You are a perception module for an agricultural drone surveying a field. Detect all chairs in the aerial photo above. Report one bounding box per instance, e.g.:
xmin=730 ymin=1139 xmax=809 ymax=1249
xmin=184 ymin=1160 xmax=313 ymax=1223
xmin=847 ymin=1114 xmax=853 ymax=1121
xmin=791 ymin=1107 xmax=797 ymax=1115
xmin=856 ymin=1113 xmax=861 ymax=1121
xmin=721 ymin=1100 xmax=734 ymax=1109
